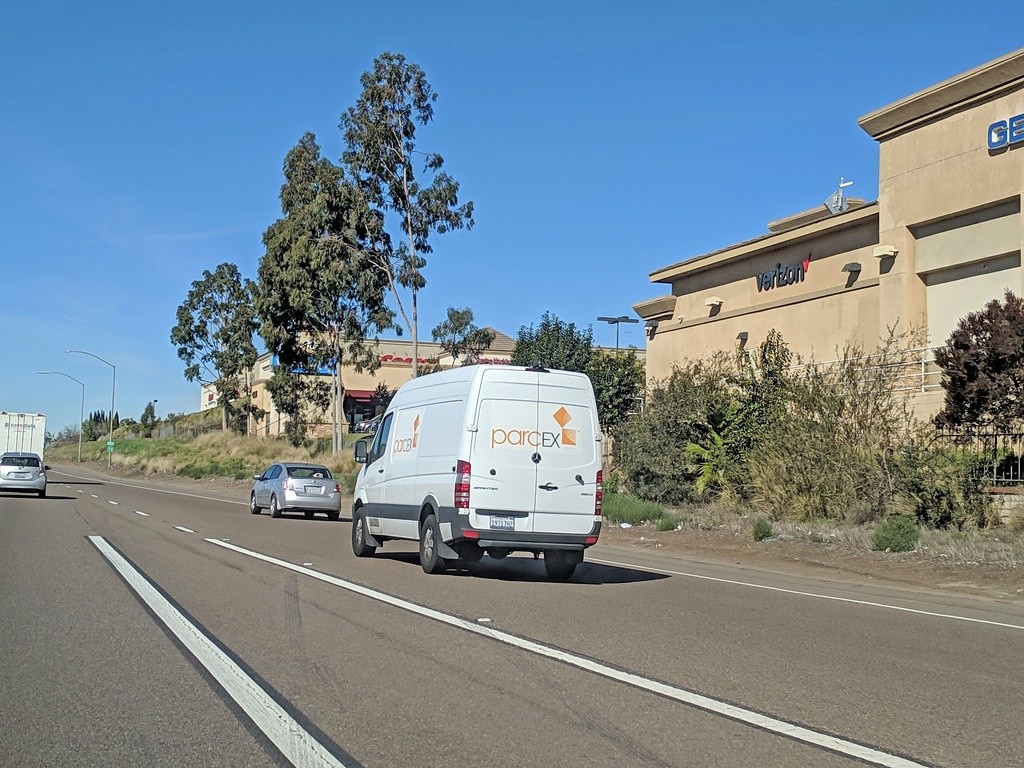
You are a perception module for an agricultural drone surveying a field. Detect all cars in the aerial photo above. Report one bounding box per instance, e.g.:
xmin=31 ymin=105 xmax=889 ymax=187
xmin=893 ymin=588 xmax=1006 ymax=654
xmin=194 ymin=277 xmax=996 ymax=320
xmin=248 ymin=462 xmax=342 ymax=519
xmin=0 ymin=453 xmax=51 ymax=496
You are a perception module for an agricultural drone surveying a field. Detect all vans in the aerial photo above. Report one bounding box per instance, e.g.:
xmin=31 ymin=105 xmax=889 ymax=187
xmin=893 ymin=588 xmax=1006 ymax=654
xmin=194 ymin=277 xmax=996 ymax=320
xmin=351 ymin=360 xmax=603 ymax=580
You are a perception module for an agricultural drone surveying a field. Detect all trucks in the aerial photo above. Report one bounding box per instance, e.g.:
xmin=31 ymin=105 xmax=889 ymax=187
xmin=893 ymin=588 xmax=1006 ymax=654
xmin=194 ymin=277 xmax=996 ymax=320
xmin=0 ymin=411 xmax=48 ymax=462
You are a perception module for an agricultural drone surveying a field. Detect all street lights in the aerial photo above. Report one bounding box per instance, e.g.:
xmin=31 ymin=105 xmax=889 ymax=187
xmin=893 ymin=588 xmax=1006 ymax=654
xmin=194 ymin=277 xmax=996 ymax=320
xmin=64 ymin=349 xmax=117 ymax=468
xmin=36 ymin=369 xmax=84 ymax=464
